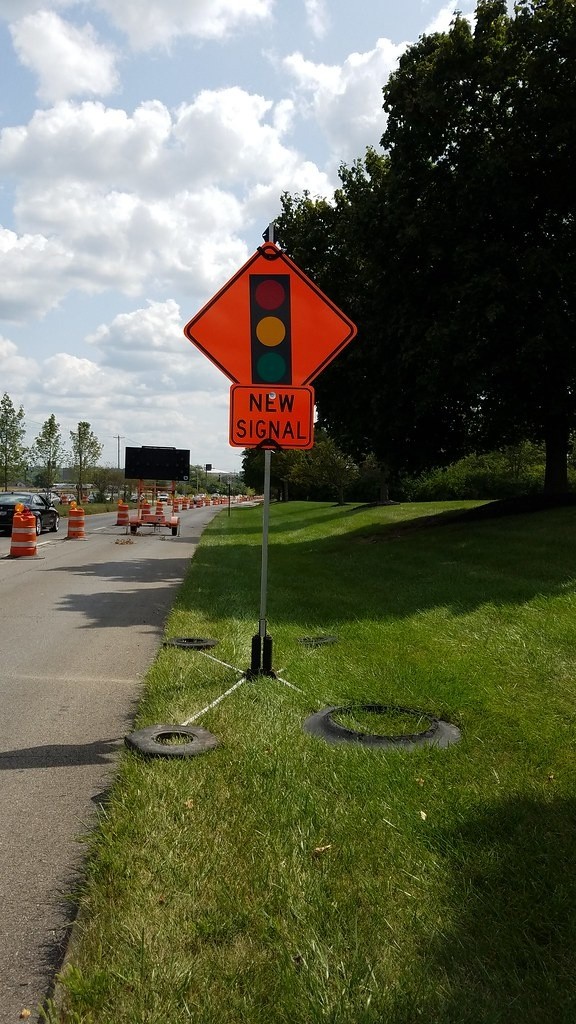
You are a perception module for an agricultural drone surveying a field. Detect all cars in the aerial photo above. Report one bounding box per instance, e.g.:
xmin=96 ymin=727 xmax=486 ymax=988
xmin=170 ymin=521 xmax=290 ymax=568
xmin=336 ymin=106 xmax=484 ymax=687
xmin=37 ymin=492 xmax=60 ymax=505
xmin=59 ymin=494 xmax=77 ymax=504
xmin=88 ymin=494 xmax=99 ymax=503
xmin=158 ymin=494 xmax=169 ymax=502
xmin=130 ymin=495 xmax=145 ymax=503
xmin=0 ymin=491 xmax=59 ymax=535
xmin=197 ymin=494 xmax=205 ymax=499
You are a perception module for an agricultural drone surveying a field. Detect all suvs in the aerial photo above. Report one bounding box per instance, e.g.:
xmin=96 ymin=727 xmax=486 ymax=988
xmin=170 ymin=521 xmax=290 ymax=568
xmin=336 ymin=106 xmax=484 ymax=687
xmin=211 ymin=494 xmax=220 ymax=500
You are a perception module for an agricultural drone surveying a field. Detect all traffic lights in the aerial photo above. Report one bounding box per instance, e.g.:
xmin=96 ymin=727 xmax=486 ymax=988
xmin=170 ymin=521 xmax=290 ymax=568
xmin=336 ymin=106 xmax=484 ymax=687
xmin=250 ymin=273 xmax=290 ymax=384
